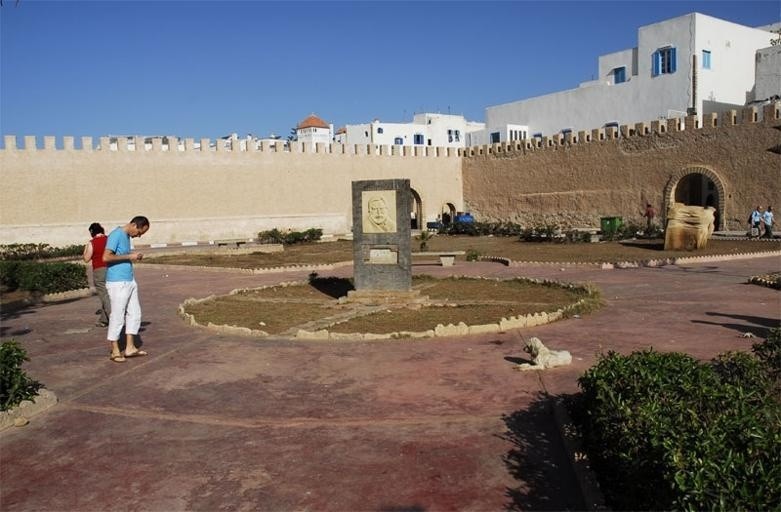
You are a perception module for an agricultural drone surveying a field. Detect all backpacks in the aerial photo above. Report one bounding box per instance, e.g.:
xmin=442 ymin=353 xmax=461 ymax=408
xmin=748 ymin=211 xmax=757 ymax=224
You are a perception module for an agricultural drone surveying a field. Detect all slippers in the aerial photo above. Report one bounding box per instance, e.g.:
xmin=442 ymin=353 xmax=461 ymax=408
xmin=112 ymin=350 xmax=147 ymax=362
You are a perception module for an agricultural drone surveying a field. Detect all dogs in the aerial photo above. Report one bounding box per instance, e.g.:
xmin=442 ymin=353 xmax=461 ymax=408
xmin=512 ymin=337 xmax=574 ymax=372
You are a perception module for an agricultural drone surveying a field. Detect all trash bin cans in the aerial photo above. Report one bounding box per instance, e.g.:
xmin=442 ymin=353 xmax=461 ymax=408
xmin=600 ymin=216 xmax=622 ymax=234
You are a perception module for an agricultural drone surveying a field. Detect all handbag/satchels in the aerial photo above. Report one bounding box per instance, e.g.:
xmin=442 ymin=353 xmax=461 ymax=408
xmin=751 ymin=228 xmax=759 ymax=236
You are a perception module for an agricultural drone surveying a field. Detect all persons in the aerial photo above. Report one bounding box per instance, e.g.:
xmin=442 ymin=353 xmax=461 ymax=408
xmin=84 ymin=221 xmax=113 ymax=328
xmin=364 ymin=194 xmax=394 ymax=233
xmin=749 ymin=206 xmax=764 ymax=240
xmin=435 ymin=214 xmax=442 ymax=227
xmin=761 ymin=206 xmax=779 ymax=240
xmin=643 ymin=205 xmax=658 ymax=229
xmin=100 ymin=214 xmax=152 ymax=365
